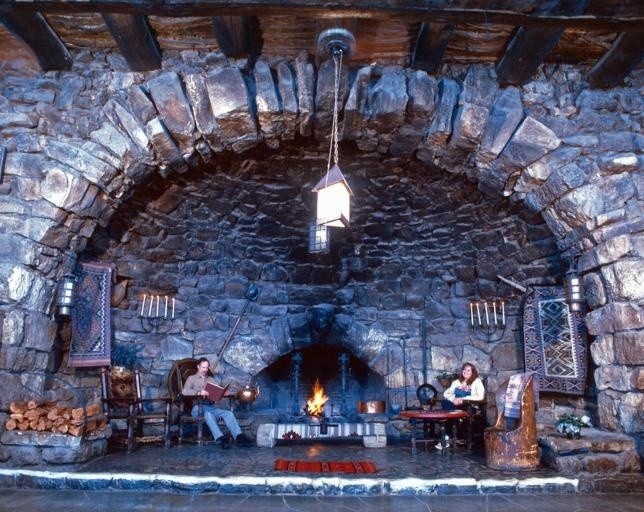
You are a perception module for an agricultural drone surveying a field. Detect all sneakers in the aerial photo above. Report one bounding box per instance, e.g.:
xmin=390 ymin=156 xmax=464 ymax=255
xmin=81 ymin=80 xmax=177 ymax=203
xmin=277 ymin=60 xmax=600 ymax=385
xmin=237 ymin=435 xmax=255 ymax=447
xmin=435 ymin=438 xmax=450 ymax=450
xmin=221 ymin=437 xmax=233 ymax=449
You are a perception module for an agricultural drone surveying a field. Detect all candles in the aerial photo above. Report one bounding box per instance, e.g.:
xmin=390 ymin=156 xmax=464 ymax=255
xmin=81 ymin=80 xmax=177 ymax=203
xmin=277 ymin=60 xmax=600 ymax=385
xmin=470 ymin=302 xmax=506 ymax=325
xmin=140 ymin=294 xmax=177 ymax=319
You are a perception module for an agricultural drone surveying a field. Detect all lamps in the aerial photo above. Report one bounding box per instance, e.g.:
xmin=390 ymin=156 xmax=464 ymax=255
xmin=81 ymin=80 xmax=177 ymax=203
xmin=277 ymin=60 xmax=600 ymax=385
xmin=55 ymin=249 xmax=76 ymax=314
xmin=562 ymin=246 xmax=587 ymax=313
xmin=311 ymin=28 xmax=356 ymax=229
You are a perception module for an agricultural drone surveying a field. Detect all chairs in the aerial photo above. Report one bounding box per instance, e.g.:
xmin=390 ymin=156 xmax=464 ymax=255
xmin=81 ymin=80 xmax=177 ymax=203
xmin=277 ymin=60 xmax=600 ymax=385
xmin=174 ymin=359 xmax=236 ymax=448
xmin=483 ymin=376 xmax=540 ymax=472
xmin=430 ymin=375 xmax=486 ymax=450
xmin=100 ymin=366 xmax=172 ymax=453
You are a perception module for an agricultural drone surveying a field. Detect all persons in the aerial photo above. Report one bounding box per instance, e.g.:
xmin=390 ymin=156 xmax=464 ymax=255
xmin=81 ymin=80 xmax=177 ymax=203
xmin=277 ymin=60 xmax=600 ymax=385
xmin=181 ymin=356 xmax=255 ymax=449
xmin=434 ymin=362 xmax=487 ymax=450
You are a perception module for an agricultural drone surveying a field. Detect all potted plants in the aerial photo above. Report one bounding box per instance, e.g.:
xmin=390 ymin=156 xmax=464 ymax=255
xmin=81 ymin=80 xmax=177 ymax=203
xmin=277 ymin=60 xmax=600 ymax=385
xmin=555 ymin=412 xmax=591 ymax=440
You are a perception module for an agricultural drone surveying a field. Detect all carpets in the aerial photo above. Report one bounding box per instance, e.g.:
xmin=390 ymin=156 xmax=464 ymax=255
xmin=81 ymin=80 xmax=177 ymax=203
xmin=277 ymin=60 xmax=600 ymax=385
xmin=275 ymin=459 xmax=377 ymax=474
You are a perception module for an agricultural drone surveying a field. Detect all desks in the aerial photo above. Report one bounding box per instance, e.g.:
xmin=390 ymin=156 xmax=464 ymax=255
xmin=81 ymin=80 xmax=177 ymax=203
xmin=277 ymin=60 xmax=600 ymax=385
xmin=257 ymin=422 xmax=388 ymax=449
xmin=399 ymin=410 xmax=468 ymax=455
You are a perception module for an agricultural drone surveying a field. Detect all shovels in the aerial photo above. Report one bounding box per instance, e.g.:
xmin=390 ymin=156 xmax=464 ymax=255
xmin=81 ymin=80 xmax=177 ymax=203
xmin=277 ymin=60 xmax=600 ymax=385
xmin=210 ymin=284 xmax=262 ymax=374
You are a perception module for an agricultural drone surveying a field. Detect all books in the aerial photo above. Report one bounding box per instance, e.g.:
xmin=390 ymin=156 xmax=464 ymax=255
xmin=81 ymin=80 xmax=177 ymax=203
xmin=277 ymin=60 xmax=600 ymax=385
xmin=203 ymin=381 xmax=231 ymax=403
